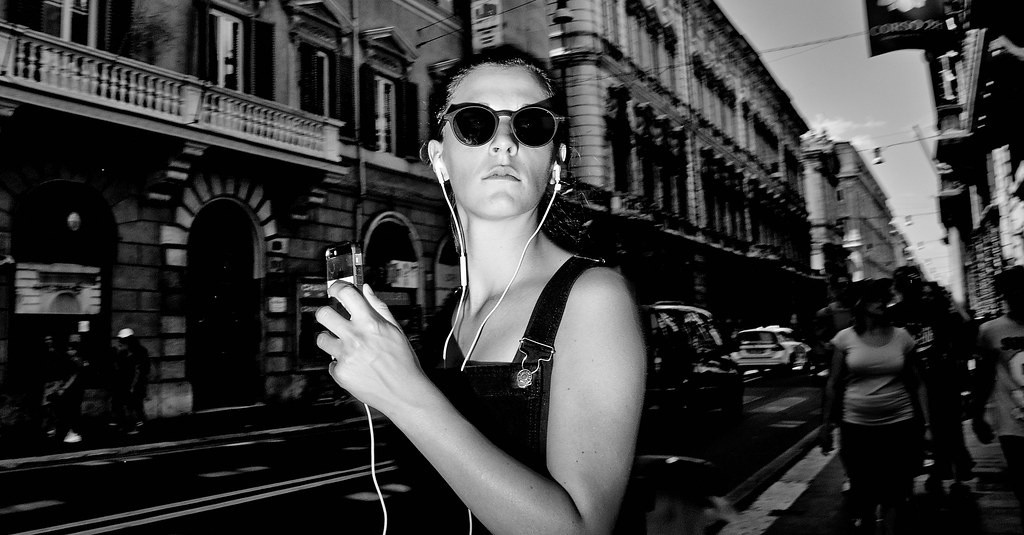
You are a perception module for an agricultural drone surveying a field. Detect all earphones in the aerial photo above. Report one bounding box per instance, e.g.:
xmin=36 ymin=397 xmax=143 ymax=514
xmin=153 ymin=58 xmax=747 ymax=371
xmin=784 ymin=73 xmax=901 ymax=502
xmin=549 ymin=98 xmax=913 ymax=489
xmin=432 ymin=152 xmax=444 ymax=184
xmin=553 ymin=161 xmax=561 ymax=182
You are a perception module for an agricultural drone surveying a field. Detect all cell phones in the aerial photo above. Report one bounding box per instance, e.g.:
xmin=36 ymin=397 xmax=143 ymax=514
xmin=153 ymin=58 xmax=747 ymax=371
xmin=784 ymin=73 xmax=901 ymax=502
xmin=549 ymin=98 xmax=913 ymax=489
xmin=324 ymin=242 xmax=364 ymax=360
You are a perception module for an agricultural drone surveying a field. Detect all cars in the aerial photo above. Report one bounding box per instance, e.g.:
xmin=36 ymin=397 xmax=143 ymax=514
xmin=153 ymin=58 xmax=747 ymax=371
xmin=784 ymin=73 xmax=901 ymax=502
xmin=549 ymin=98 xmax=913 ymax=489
xmin=730 ymin=325 xmax=816 ymax=373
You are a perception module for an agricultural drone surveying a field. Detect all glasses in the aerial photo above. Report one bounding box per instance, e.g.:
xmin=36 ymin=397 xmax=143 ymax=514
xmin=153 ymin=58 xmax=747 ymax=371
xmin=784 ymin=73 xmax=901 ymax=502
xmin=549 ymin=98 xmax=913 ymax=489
xmin=436 ymin=105 xmax=565 ymax=148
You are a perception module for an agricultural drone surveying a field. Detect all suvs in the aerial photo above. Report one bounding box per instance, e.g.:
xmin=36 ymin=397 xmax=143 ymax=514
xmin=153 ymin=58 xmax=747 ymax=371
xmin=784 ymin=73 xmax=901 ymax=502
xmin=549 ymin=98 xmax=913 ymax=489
xmin=638 ymin=298 xmax=745 ymax=423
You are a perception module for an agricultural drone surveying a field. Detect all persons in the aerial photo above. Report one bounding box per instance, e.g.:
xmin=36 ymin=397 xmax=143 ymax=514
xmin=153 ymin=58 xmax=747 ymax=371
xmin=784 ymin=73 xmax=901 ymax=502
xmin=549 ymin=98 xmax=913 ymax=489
xmin=0 ymin=323 xmax=151 ymax=448
xmin=313 ymin=43 xmax=646 ymax=535
xmin=817 ymin=262 xmax=1023 ymax=535
